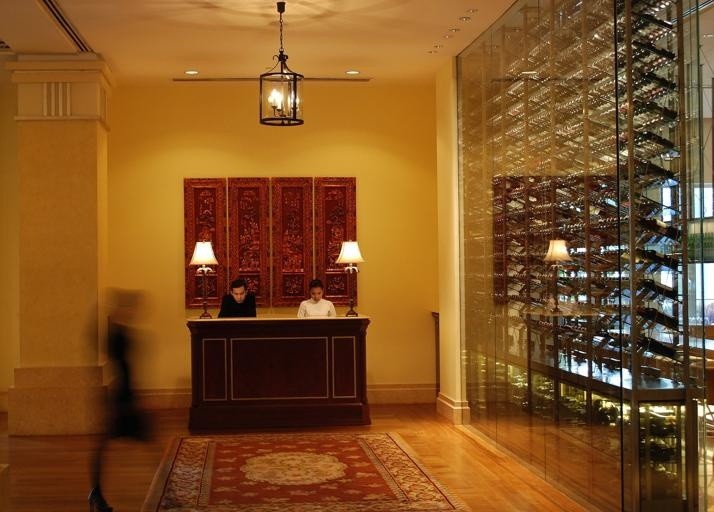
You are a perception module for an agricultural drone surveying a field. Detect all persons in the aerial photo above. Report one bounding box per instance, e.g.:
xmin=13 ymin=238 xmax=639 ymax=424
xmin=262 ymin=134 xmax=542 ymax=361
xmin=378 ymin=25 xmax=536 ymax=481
xmin=295 ymin=278 xmax=337 ymax=317
xmin=84 ymin=284 xmax=191 ymax=512
xmin=217 ymin=277 xmax=257 ymax=318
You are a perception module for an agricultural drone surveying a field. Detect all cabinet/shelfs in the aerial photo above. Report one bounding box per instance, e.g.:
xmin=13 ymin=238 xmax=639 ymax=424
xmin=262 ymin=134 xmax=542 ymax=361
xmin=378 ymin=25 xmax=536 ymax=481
xmin=451 ymin=0 xmax=700 ymax=387
xmin=468 ymin=347 xmax=708 ymax=511
xmin=184 ymin=312 xmax=371 ymax=437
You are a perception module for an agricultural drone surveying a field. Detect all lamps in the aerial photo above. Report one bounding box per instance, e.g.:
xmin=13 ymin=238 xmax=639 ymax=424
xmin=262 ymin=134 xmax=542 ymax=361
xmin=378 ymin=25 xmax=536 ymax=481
xmin=254 ymin=0 xmax=309 ymax=128
xmin=333 ymin=238 xmax=366 ymax=316
xmin=187 ymin=239 xmax=219 ymax=319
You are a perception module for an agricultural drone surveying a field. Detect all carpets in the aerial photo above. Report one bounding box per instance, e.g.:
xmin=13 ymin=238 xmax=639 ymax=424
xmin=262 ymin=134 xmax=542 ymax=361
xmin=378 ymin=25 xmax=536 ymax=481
xmin=138 ymin=430 xmax=471 ymax=512
